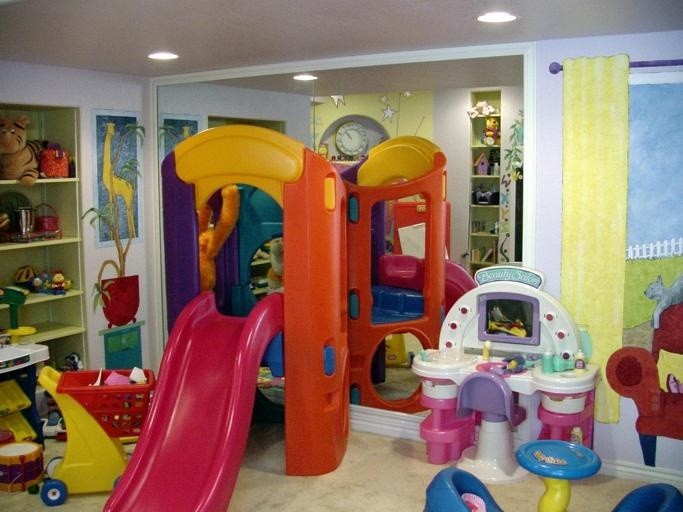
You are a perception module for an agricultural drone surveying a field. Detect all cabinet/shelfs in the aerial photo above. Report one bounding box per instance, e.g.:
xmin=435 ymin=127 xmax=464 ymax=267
xmin=435 ymin=84 xmax=516 ymax=287
xmin=0 ymin=103 xmax=90 ymax=376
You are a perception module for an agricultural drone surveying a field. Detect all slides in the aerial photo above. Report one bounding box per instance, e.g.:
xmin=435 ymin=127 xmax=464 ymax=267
xmin=102 ymin=293 xmax=285 ymax=511
xmin=378 ymin=254 xmax=478 ymax=300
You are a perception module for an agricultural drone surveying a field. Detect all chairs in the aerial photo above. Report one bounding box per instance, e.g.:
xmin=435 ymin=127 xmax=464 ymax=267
xmin=422 ymin=373 xmax=683 ymax=511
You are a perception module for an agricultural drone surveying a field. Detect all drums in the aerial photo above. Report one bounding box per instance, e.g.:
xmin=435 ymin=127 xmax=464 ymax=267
xmin=0 ymin=442 xmax=46 ymax=493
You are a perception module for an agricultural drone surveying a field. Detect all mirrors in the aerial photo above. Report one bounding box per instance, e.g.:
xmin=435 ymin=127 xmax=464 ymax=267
xmin=145 ymin=43 xmax=537 ymax=443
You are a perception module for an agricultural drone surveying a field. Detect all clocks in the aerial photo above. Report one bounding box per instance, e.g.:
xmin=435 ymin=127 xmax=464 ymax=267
xmin=334 ymin=122 xmax=369 ymax=158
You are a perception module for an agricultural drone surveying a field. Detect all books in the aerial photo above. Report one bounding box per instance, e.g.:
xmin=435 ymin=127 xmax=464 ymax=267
xmin=491 ymin=238 xmax=498 ymax=265
